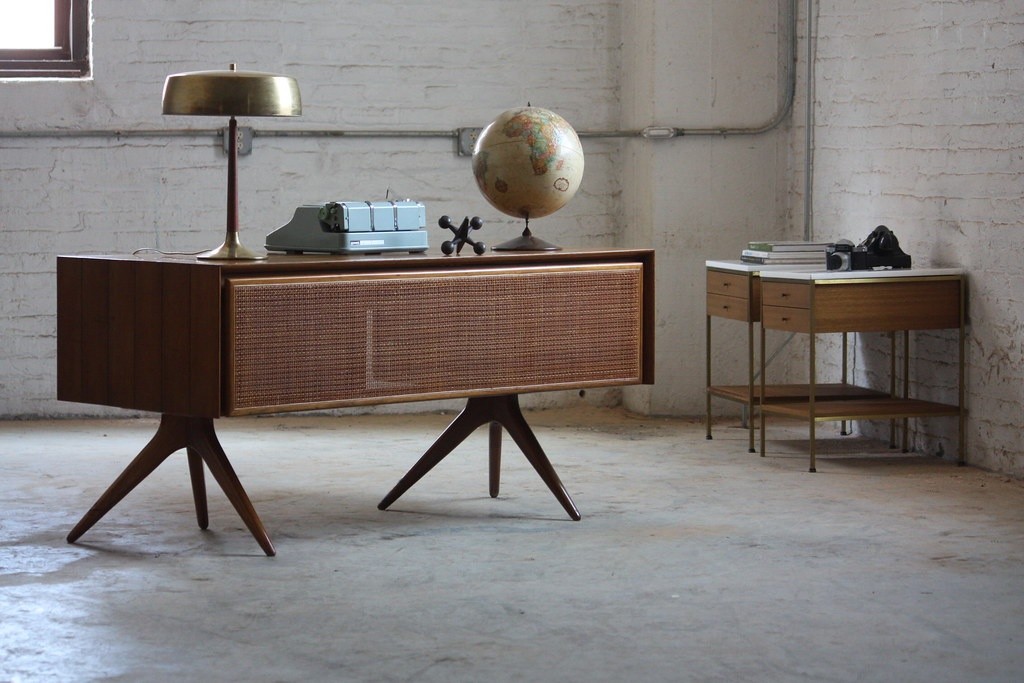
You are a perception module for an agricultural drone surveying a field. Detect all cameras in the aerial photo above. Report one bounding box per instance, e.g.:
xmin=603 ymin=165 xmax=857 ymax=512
xmin=825 ymin=244 xmax=871 ymax=272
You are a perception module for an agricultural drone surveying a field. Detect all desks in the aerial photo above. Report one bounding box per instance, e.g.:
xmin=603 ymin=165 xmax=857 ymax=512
xmin=759 ymin=266 xmax=971 ymax=472
xmin=54 ymin=247 xmax=658 ymax=560
xmin=704 ymin=259 xmax=897 ymax=453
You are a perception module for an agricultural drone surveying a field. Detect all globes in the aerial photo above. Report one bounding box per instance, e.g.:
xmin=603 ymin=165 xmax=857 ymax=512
xmin=470 ymin=101 xmax=586 ymax=253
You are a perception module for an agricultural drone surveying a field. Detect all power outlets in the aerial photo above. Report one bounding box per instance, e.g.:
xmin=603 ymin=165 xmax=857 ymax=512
xmin=222 ymin=126 xmax=253 ymax=156
xmin=459 ymin=126 xmax=483 ymax=154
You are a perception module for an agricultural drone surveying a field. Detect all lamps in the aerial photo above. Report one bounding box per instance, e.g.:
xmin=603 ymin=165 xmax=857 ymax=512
xmin=161 ymin=63 xmax=304 ymax=260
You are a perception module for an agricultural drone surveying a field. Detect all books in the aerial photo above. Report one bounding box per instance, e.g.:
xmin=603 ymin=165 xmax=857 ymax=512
xmin=740 ymin=239 xmax=828 ymax=265
